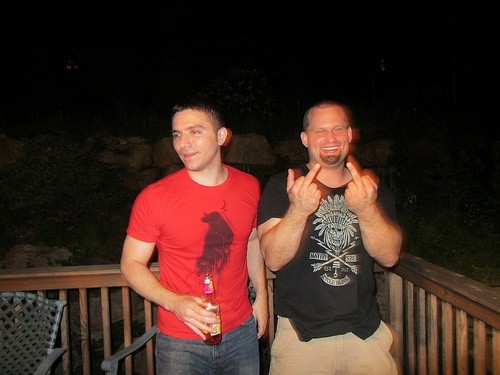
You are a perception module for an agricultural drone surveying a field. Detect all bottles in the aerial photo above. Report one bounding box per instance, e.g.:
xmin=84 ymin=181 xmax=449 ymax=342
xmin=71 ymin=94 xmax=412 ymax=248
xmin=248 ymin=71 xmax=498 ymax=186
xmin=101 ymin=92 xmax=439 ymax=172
xmin=199 ymin=272 xmax=223 ymax=347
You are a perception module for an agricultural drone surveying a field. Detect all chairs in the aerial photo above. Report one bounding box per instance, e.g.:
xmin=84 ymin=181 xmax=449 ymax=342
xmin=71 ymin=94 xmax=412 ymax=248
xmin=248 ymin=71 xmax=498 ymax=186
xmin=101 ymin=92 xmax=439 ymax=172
xmin=0 ymin=291 xmax=67 ymax=375
xmin=101 ymin=324 xmax=158 ymax=375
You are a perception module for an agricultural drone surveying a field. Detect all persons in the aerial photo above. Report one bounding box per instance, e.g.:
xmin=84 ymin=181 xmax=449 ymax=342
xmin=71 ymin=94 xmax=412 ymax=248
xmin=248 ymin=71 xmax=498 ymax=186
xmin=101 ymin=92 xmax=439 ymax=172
xmin=120 ymin=95 xmax=268 ymax=375
xmin=258 ymin=98 xmax=403 ymax=375
